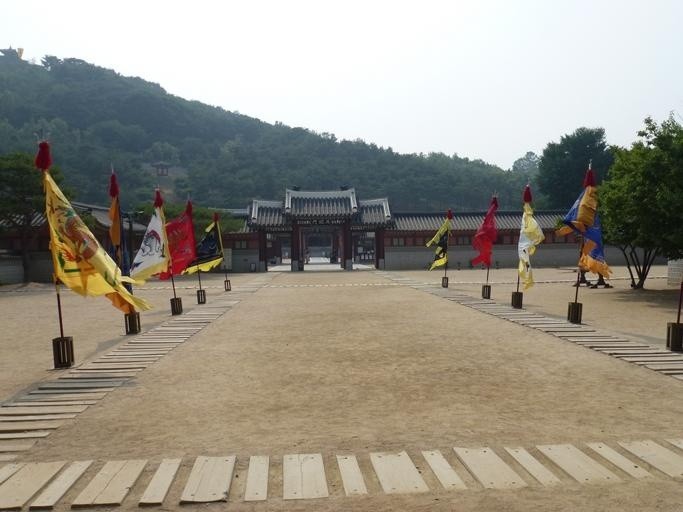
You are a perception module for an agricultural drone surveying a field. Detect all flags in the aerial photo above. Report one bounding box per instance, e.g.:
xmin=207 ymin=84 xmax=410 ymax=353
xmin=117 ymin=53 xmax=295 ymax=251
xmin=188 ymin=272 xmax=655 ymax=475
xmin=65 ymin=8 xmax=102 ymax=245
xmin=181 ymin=222 xmax=224 ymax=274
xmin=130 ymin=204 xmax=171 ymax=280
xmin=555 ymin=184 xmax=613 ymax=280
xmin=518 ymin=203 xmax=546 ymax=291
xmin=471 ymin=203 xmax=498 ymax=268
xmin=160 ymin=210 xmax=196 ymax=280
xmin=108 ymin=196 xmax=132 ymax=293
xmin=426 ymin=218 xmax=452 ymax=272
xmin=44 ymin=172 xmax=154 ymax=315
